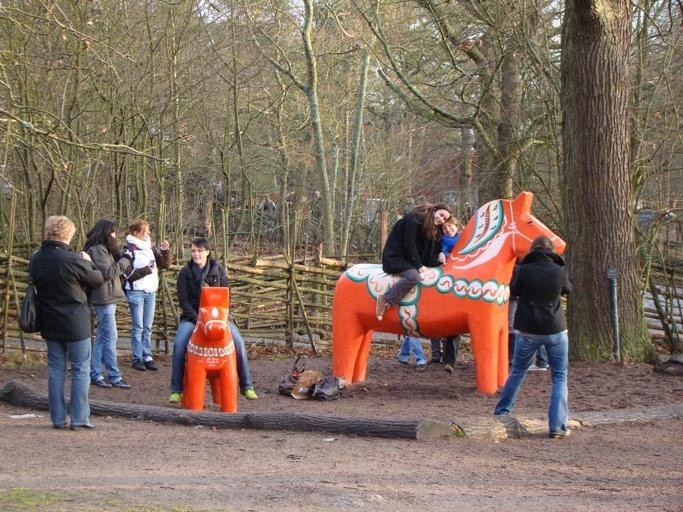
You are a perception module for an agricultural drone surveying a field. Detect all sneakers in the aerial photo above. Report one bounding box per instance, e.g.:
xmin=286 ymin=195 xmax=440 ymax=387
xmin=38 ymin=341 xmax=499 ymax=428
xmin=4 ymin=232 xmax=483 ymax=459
xmin=399 ymin=351 xmax=454 ymax=376
xmin=72 ymin=424 xmax=95 ymax=430
xmin=376 ymin=295 xmax=389 ymax=320
xmin=92 ymin=380 xmax=112 ymax=387
xmin=240 ymin=387 xmax=258 ymax=399
xmin=169 ymin=393 xmax=180 ymax=403
xmin=109 ymin=380 xmax=132 ymax=389
xmin=53 ymin=423 xmax=69 ymax=430
xmin=144 ymin=361 xmax=158 ymax=370
xmin=133 ymin=363 xmax=146 ymax=371
xmin=549 ymin=429 xmax=572 ymax=438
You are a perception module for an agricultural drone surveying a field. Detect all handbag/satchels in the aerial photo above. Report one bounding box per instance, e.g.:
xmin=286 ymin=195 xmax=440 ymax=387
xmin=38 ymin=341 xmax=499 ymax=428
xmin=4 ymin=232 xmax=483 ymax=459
xmin=280 ymin=370 xmax=346 ymax=399
xmin=19 ymin=284 xmax=40 ymax=333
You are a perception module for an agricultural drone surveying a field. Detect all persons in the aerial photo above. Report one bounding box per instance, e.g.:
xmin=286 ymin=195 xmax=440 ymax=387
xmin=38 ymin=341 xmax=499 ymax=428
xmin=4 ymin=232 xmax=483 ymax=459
xmin=396 ymin=333 xmax=427 ymax=371
xmin=492 ymin=233 xmax=574 ymax=439
xmin=508 ymin=298 xmax=550 ymax=372
xmin=427 ymin=216 xmax=465 ymax=375
xmin=375 ymin=202 xmax=453 ymax=322
xmin=83 ymin=217 xmax=135 ymax=389
xmin=257 ymin=191 xmax=279 ymax=211
xmin=31 ymin=213 xmax=103 ymax=432
xmin=167 ymin=238 xmax=258 ymax=404
xmin=310 ymin=190 xmax=323 ymax=218
xmin=119 ymin=217 xmax=172 ymax=370
